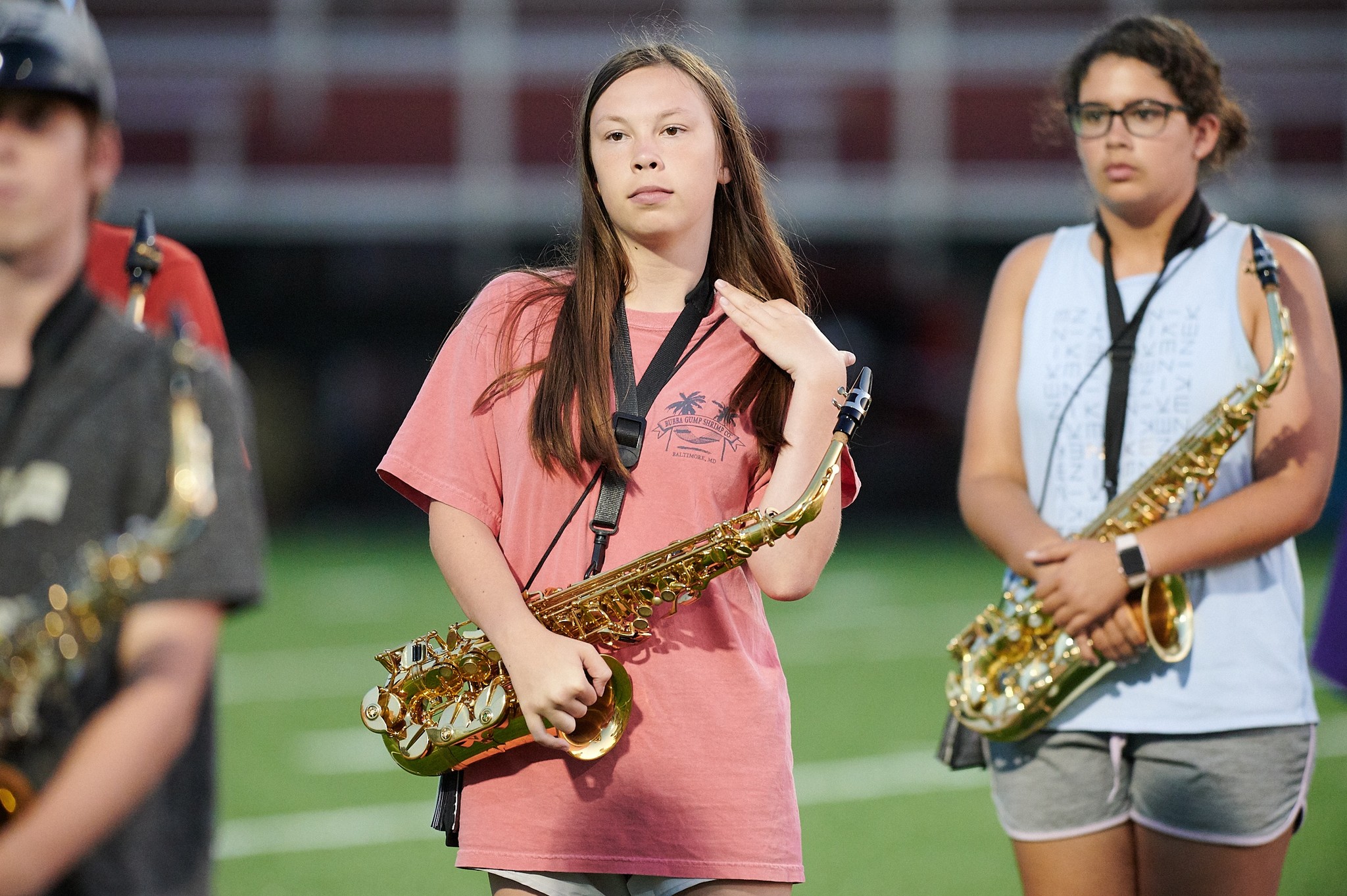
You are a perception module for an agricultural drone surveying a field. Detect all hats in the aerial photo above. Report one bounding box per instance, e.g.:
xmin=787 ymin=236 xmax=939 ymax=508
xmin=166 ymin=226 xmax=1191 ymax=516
xmin=0 ymin=0 xmax=122 ymax=117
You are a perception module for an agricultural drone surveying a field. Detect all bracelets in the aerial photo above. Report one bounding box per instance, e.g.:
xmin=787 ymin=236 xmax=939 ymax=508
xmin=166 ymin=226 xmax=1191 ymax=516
xmin=1115 ymin=533 xmax=1148 ymax=591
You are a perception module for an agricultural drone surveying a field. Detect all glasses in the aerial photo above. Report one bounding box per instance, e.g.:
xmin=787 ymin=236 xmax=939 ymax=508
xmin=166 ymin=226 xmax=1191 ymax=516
xmin=1069 ymin=98 xmax=1193 ymax=138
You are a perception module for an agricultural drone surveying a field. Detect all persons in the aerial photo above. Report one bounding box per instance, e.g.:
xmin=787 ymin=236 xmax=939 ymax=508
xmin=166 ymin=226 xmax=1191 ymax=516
xmin=957 ymin=16 xmax=1342 ymax=896
xmin=85 ymin=222 xmax=228 ymax=355
xmin=377 ymin=42 xmax=862 ymax=896
xmin=0 ymin=0 xmax=265 ymax=896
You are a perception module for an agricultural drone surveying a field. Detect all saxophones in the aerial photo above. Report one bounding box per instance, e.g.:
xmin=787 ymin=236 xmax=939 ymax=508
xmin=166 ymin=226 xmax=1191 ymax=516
xmin=943 ymin=220 xmax=1297 ymax=742
xmin=0 ymin=308 xmax=222 ymax=830
xmin=358 ymin=365 xmax=875 ymax=778
